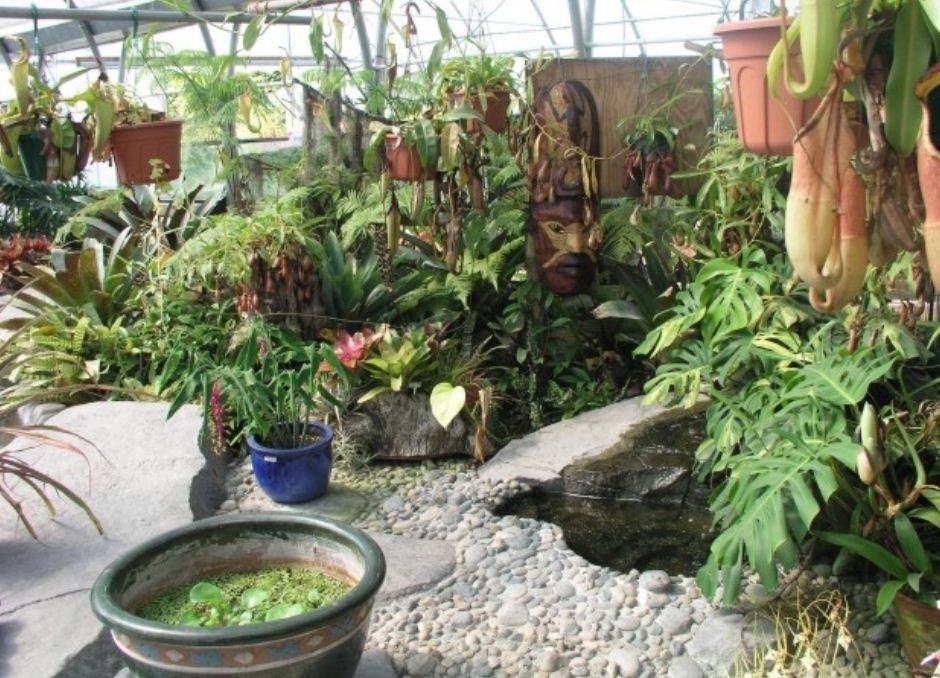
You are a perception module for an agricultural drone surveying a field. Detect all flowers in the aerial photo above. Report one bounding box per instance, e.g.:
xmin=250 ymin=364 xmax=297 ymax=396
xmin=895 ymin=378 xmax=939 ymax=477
xmin=331 ymin=330 xmax=372 ymax=368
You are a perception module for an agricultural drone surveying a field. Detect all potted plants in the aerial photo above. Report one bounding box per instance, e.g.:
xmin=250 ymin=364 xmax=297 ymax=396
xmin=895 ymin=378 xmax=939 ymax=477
xmin=104 ymin=79 xmax=185 ymax=186
xmin=91 ymin=513 xmax=385 ymax=678
xmin=153 ymin=319 xmax=370 ymax=505
xmin=381 ymin=88 xmax=430 ymax=180
xmin=0 ymin=33 xmax=123 ymax=182
xmin=441 ymin=55 xmax=518 ymax=130
xmin=714 ymin=0 xmax=834 ymax=155
